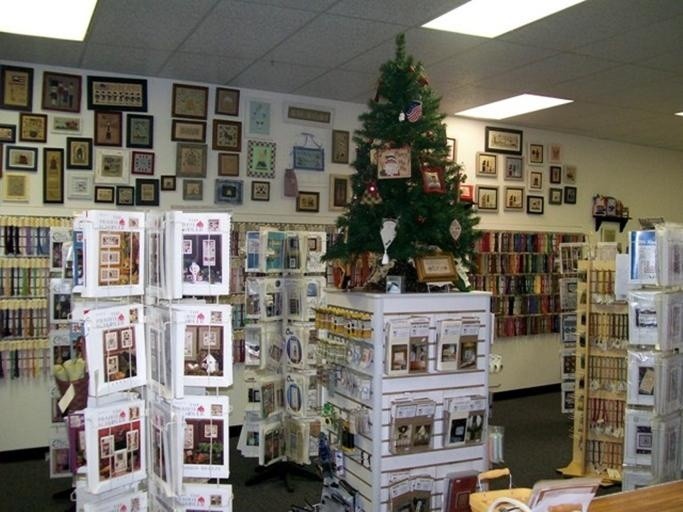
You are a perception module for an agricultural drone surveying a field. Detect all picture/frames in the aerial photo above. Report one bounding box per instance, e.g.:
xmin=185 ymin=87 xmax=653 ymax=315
xmin=414 ymin=250 xmax=459 ymax=285
xmin=437 ymin=125 xmax=578 ymax=215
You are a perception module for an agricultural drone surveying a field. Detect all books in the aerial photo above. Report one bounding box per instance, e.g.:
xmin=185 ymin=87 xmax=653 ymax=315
xmin=47 ymin=209 xmax=147 ymax=510
xmin=527 ymin=475 xmax=602 ymax=512
xmin=383 ymin=314 xmax=488 ymax=511
xmin=145 ymin=210 xmax=233 ymax=512
